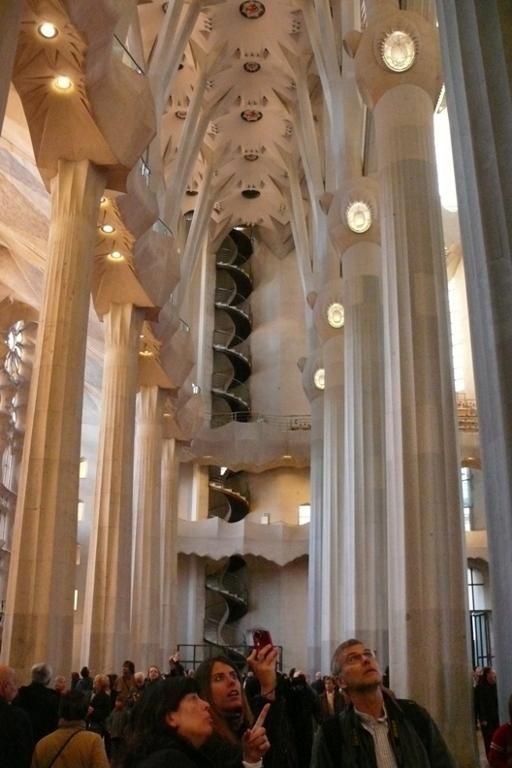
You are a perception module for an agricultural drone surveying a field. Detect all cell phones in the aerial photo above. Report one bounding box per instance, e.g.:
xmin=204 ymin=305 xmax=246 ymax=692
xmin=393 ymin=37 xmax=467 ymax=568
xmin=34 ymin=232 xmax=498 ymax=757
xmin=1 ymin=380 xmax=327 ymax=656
xmin=253 ymin=630 xmax=274 ymax=660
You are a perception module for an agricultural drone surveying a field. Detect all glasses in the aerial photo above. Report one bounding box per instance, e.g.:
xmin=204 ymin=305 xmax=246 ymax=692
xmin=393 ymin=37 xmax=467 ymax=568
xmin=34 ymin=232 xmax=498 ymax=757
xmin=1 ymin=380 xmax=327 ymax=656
xmin=336 ymin=650 xmax=377 ymax=675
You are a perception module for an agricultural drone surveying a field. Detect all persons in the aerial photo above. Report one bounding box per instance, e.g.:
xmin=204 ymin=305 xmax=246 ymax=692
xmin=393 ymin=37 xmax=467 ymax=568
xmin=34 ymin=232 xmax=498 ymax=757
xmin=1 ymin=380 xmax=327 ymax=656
xmin=473 ymin=666 xmax=484 ymax=728
xmin=477 ymin=667 xmax=499 ymax=765
xmin=487 ymin=694 xmax=512 ymax=768
xmin=1 ymin=640 xmax=454 ymax=768
